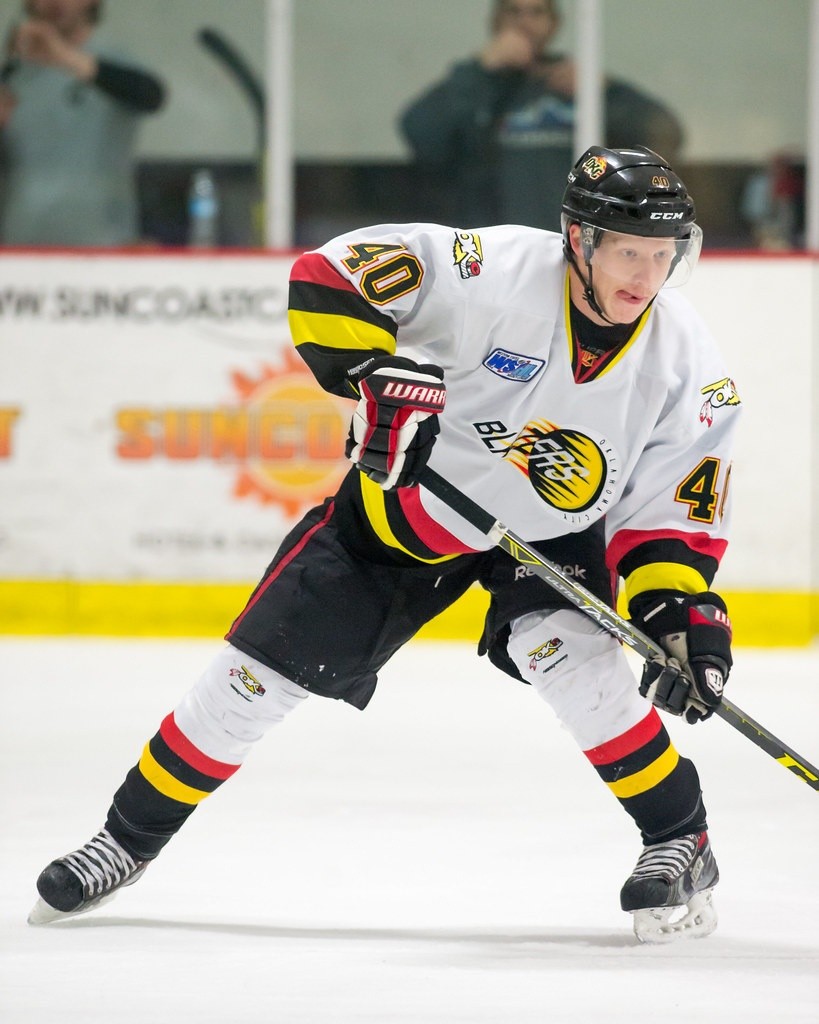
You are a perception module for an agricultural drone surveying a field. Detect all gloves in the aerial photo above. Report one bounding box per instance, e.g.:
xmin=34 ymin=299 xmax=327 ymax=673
xmin=638 ymin=591 xmax=733 ymax=725
xmin=345 ymin=367 xmax=447 ymax=492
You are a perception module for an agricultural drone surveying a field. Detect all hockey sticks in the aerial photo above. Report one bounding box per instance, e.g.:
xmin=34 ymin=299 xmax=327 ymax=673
xmin=200 ymin=24 xmax=267 ymax=247
xmin=417 ymin=465 xmax=819 ymax=797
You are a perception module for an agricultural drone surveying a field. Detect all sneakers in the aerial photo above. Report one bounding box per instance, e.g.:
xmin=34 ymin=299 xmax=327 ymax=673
xmin=26 ymin=827 xmax=151 ymax=925
xmin=620 ymin=830 xmax=719 ymax=943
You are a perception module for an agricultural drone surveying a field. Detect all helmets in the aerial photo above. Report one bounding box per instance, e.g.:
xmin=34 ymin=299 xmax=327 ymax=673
xmin=560 ymin=145 xmax=697 ymax=256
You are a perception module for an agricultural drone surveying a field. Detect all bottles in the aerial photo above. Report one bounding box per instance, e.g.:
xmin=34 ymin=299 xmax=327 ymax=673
xmin=185 ymin=168 xmax=221 ymax=248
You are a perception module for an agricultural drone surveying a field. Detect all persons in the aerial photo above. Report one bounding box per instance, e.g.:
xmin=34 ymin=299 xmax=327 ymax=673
xmin=400 ymin=0 xmax=685 ymax=246
xmin=32 ymin=145 xmax=741 ymax=944
xmin=1 ymin=1 xmax=169 ymax=247
